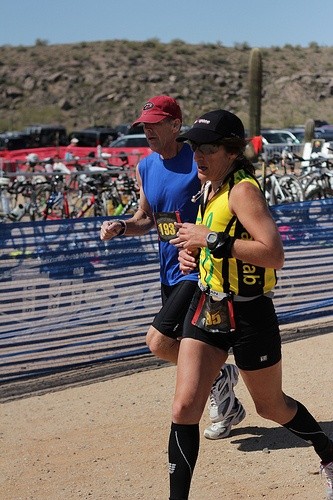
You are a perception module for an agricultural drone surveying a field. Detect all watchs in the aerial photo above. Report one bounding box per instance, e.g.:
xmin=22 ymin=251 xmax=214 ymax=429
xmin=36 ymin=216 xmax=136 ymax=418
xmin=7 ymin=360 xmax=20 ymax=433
xmin=207 ymin=230 xmax=219 ymax=252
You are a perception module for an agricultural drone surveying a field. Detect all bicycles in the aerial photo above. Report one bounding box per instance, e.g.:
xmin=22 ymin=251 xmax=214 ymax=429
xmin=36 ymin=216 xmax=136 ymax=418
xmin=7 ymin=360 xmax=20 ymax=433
xmin=256 ymin=146 xmax=333 ymax=224
xmin=0 ymin=152 xmax=160 ymax=269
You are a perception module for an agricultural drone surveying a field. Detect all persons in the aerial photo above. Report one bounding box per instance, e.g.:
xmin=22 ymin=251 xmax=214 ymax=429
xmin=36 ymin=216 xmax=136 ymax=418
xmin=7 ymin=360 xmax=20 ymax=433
xmin=166 ymin=108 xmax=332 ymax=500
xmin=97 ymin=94 xmax=247 ymax=441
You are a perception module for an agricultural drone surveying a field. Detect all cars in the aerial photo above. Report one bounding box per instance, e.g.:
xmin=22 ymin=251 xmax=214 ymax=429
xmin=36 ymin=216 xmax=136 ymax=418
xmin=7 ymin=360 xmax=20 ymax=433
xmin=0 ymin=123 xmax=192 ymax=192
xmin=244 ymin=120 xmax=333 ymax=165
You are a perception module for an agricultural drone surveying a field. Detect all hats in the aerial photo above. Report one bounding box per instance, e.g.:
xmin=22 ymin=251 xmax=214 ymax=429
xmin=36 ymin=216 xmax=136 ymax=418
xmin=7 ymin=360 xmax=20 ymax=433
xmin=175 ymin=110 xmax=245 ymax=144
xmin=131 ymin=96 xmax=181 ymax=127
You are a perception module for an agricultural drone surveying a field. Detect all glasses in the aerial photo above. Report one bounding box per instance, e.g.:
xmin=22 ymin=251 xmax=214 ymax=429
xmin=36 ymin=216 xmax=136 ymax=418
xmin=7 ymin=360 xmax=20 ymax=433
xmin=189 ymin=140 xmax=222 ymax=155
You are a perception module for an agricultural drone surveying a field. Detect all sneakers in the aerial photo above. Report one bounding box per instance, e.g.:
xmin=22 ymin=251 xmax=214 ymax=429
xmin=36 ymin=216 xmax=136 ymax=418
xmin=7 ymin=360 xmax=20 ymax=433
xmin=320 ymin=461 xmax=332 ymax=500
xmin=208 ymin=363 xmax=239 ymax=423
xmin=203 ymin=399 xmax=245 ymax=440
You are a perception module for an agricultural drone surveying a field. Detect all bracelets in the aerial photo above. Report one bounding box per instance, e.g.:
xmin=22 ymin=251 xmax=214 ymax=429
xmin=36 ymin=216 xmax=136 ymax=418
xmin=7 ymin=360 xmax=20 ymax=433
xmin=111 ymin=218 xmax=127 ymax=237
xmin=212 ymin=231 xmax=236 ymax=260
